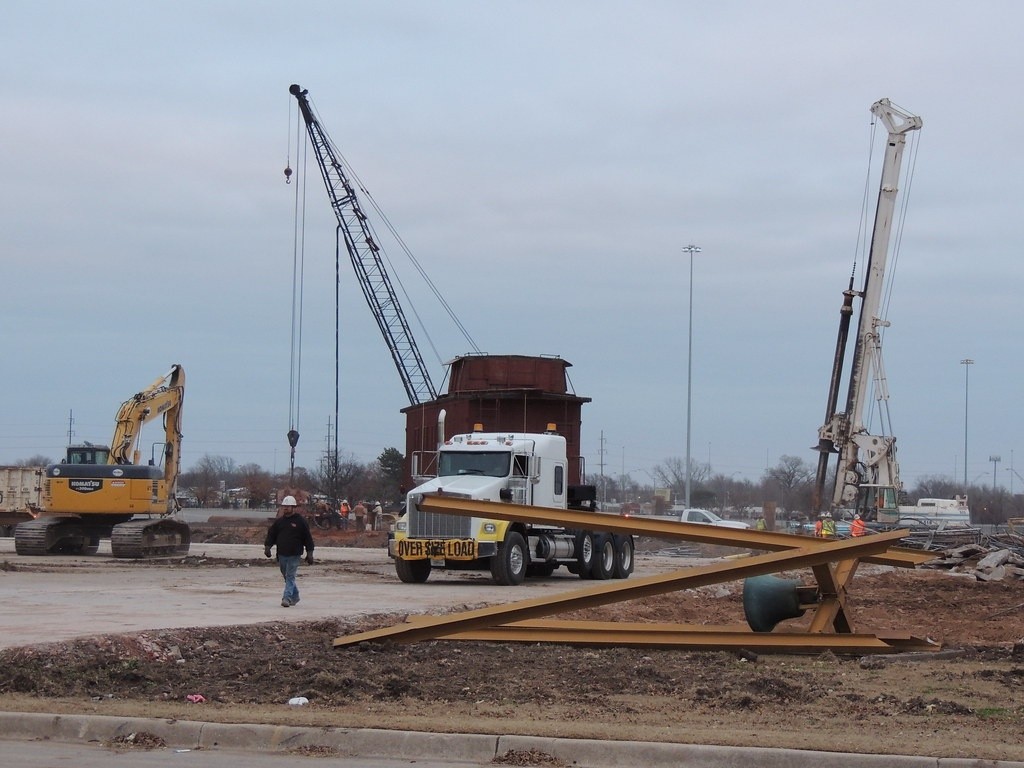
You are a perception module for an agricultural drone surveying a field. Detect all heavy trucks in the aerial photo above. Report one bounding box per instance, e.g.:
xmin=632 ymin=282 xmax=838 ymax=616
xmin=387 ymin=432 xmax=636 ymax=586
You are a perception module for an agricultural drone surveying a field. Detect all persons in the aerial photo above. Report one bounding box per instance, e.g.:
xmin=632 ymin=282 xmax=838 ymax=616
xmin=264 ymin=496 xmax=314 ymax=607
xmin=820 ymin=512 xmax=836 ymax=538
xmin=340 ymin=500 xmax=351 ymax=529
xmin=502 ymin=456 xmax=521 ymax=475
xmin=372 ymin=502 xmax=383 ymax=531
xmin=849 ymin=514 xmax=866 ymax=537
xmin=351 ymin=501 xmax=366 ymax=531
xmin=815 ymin=519 xmax=822 ymax=536
xmin=755 ymin=515 xmax=768 ymax=530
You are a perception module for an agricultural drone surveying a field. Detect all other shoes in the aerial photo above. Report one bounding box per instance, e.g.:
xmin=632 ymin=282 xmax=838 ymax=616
xmin=281 ymin=597 xmax=290 ymax=607
xmin=290 ymin=596 xmax=300 ymax=606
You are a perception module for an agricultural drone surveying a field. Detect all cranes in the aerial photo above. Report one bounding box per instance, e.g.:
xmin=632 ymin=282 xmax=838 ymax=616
xmin=286 ymin=82 xmax=599 ymax=516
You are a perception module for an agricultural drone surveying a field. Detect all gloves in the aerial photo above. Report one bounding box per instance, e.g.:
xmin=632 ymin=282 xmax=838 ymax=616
xmin=264 ymin=546 xmax=272 ymax=558
xmin=305 ymin=551 xmax=313 ymax=565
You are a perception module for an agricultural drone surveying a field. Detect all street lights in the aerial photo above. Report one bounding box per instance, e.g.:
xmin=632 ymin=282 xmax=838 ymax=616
xmin=960 ymin=359 xmax=975 ymax=494
xmin=989 ymin=456 xmax=1001 ymax=523
xmin=682 ymin=244 xmax=702 ymax=509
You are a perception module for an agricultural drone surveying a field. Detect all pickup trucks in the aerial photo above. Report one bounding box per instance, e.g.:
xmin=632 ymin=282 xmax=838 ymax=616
xmin=623 ymin=508 xmax=750 ymax=530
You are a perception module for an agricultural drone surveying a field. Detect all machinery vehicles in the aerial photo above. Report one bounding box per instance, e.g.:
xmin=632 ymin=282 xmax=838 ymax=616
xmin=14 ymin=362 xmax=191 ymax=559
xmin=806 ymin=93 xmax=924 ymax=537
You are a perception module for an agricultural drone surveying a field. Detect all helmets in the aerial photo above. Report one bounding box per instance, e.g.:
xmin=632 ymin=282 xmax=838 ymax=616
xmin=343 ymin=500 xmax=347 ymax=503
xmin=375 ymin=501 xmax=380 ymax=505
xmin=821 ymin=513 xmax=825 ymax=517
xmin=281 ymin=496 xmax=297 ymax=506
xmin=826 ymin=513 xmax=832 ymax=517
xmin=854 ymin=514 xmax=860 ymax=520
